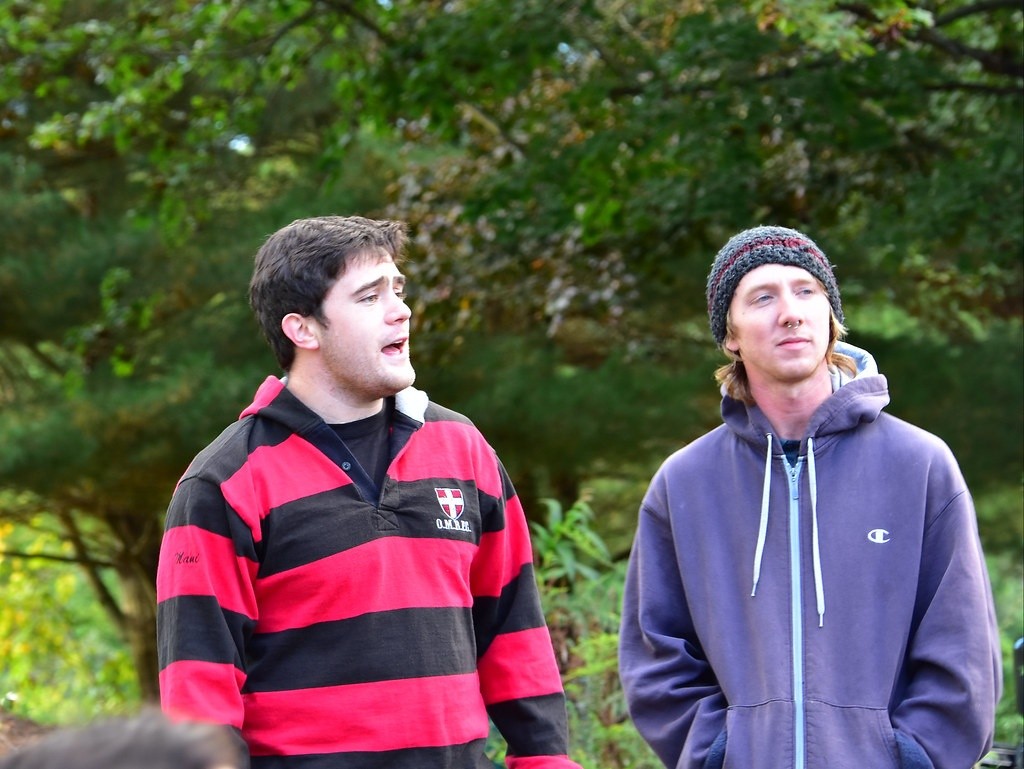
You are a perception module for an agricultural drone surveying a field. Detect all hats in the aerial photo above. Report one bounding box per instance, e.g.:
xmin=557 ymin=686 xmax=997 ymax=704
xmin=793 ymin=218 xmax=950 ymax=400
xmin=706 ymin=225 xmax=844 ymax=343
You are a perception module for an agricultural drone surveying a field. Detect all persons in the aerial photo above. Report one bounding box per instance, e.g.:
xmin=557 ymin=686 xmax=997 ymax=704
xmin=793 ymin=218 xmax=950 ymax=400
xmin=618 ymin=224 xmax=1003 ymax=769
xmin=156 ymin=215 xmax=583 ymax=769
